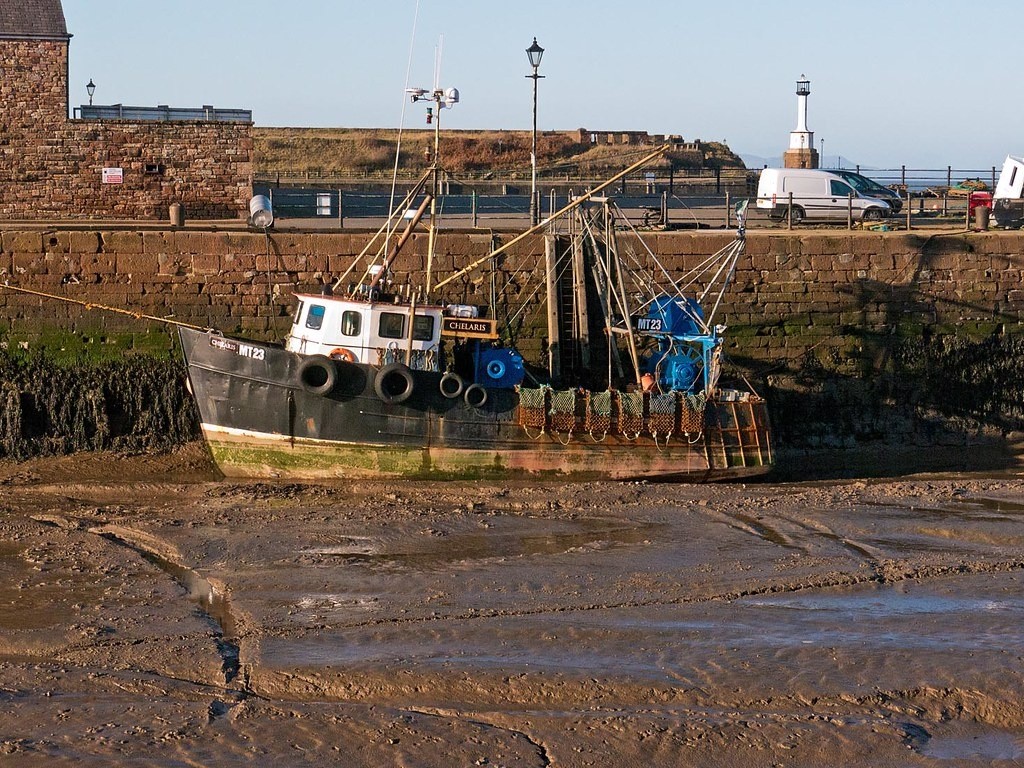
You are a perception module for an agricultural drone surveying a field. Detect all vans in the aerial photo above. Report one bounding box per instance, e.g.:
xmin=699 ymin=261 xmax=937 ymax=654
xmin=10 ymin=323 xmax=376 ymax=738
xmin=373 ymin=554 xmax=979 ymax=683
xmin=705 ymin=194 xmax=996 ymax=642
xmin=812 ymin=169 xmax=903 ymax=215
xmin=757 ymin=168 xmax=893 ymax=225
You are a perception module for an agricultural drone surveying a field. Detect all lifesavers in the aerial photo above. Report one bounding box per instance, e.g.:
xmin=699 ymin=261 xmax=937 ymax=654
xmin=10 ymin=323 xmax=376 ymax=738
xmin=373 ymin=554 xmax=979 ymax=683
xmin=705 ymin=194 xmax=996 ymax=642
xmin=328 ymin=348 xmax=355 ymax=362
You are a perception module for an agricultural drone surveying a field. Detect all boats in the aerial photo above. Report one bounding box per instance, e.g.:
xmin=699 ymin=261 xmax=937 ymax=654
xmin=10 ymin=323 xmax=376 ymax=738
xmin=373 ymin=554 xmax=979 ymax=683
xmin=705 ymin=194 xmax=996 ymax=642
xmin=177 ymin=35 xmax=775 ymax=478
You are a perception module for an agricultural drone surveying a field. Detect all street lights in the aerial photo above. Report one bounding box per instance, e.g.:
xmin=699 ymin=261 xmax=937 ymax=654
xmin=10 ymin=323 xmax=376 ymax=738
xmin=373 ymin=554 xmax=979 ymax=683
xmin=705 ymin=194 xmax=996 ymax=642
xmin=86 ymin=78 xmax=97 ymax=103
xmin=524 ymin=37 xmax=546 ymax=229
